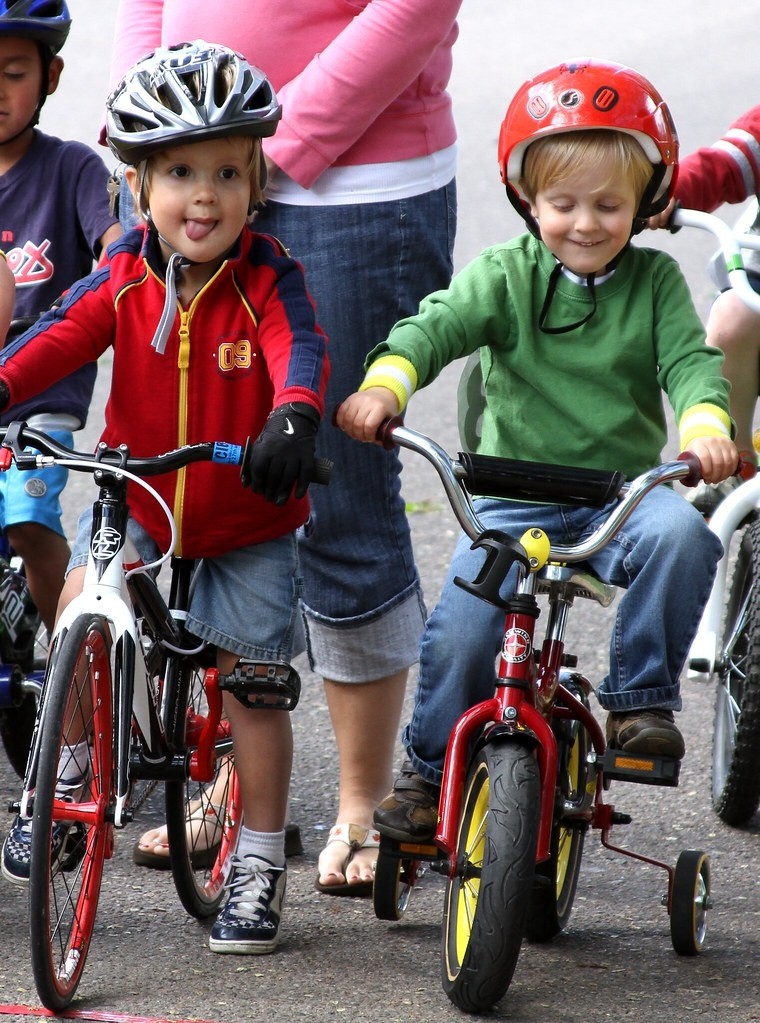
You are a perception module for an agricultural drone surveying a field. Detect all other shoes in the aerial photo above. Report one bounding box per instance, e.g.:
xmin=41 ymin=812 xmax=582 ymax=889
xmin=682 ymin=451 xmax=760 ymax=525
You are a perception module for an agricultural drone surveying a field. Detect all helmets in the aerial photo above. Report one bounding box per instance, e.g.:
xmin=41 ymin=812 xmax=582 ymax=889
xmin=497 ymin=58 xmax=679 ymax=219
xmin=107 ymin=39 xmax=282 ymax=164
xmin=0 ymin=0 xmax=72 ymax=54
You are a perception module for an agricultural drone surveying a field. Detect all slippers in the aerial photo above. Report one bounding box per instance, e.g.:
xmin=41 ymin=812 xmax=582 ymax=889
xmin=131 ymin=799 xmax=302 ymax=868
xmin=317 ymin=824 xmax=381 ymax=892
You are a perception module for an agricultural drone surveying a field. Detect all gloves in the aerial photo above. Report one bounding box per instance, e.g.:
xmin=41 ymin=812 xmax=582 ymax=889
xmin=241 ymin=402 xmax=319 ymax=504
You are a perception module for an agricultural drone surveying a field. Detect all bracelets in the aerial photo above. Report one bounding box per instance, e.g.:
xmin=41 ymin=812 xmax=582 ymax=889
xmin=677 ymin=402 xmax=732 ymax=451
xmin=358 ymin=354 xmax=418 ymax=416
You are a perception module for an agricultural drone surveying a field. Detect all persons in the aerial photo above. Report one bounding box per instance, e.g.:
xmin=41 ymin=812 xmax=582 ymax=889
xmin=0 ymin=38 xmax=330 ymax=956
xmin=115 ymin=1 xmax=465 ymax=895
xmin=336 ymin=62 xmax=739 ymax=847
xmin=643 ymin=104 xmax=760 ymax=522
xmin=0 ymin=1 xmax=123 ymax=650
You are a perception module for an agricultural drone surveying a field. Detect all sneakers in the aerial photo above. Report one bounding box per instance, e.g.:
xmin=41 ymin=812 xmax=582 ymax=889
xmin=371 ymin=761 xmax=439 ymax=842
xmin=606 ymin=708 xmax=685 ymax=761
xmin=209 ymin=854 xmax=286 ymax=952
xmin=2 ymin=756 xmax=92 ymax=885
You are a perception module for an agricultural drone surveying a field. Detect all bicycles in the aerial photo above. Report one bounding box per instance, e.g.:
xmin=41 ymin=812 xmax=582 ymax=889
xmin=330 ymin=401 xmax=760 ymax=1016
xmin=1 ymin=420 xmax=244 ymax=1010
